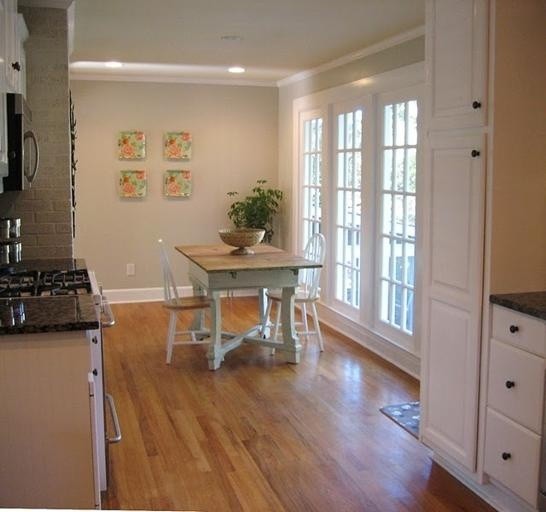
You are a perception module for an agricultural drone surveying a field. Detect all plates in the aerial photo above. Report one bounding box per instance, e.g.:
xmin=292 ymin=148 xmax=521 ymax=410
xmin=162 ymin=131 xmax=191 ymax=161
xmin=163 ymin=168 xmax=192 ymax=197
xmin=118 ymin=169 xmax=145 ymax=199
xmin=117 ymin=131 xmax=146 ymax=160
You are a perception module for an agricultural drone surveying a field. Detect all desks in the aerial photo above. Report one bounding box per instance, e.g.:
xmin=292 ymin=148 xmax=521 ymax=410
xmin=173 ymin=240 xmax=323 ymax=370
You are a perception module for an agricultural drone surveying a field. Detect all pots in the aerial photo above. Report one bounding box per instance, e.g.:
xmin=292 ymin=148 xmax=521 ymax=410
xmin=0 ymin=216 xmax=22 ymax=241
xmin=0 ymin=300 xmax=26 ymax=329
xmin=0 ymin=241 xmax=21 ymax=269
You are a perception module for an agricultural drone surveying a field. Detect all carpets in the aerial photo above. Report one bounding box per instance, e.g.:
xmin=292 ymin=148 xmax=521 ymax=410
xmin=379 ymin=399 xmax=420 ymax=440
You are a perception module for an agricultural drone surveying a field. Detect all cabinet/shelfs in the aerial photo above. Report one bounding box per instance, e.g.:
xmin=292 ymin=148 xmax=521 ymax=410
xmin=1 ymin=331 xmax=102 ymax=508
xmin=484 ymin=291 xmax=546 ymax=512
xmin=414 ymin=0 xmax=545 ymax=472
xmin=0 ymin=1 xmax=31 ymax=100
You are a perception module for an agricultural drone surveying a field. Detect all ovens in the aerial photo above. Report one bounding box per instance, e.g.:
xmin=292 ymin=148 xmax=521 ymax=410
xmin=89 ymin=298 xmax=121 ymax=491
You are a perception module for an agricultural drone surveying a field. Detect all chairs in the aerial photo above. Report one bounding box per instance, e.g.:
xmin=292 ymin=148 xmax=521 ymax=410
xmin=262 ymin=232 xmax=326 ymax=356
xmin=156 ymin=239 xmax=219 ymax=364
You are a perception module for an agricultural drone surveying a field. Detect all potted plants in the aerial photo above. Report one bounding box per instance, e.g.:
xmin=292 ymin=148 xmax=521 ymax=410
xmin=219 ymin=177 xmax=284 ymax=256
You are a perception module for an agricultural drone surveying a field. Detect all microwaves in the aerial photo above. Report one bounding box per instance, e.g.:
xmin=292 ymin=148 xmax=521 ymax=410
xmin=2 ymin=93 xmax=37 ymax=191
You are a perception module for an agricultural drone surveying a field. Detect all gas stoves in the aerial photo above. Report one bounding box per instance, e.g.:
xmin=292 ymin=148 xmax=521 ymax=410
xmin=0 ymin=270 xmax=90 ymax=298
